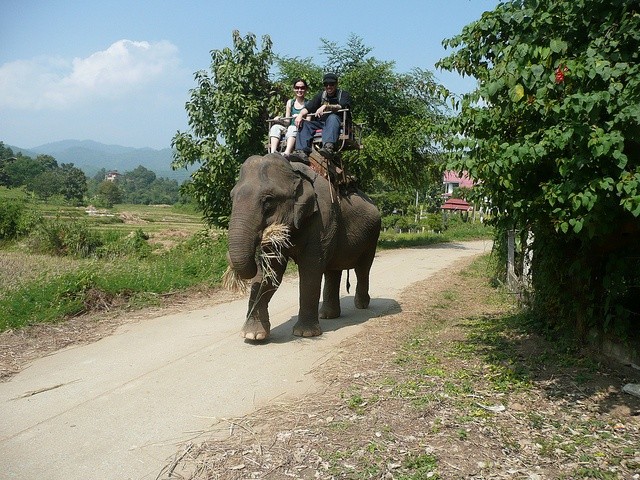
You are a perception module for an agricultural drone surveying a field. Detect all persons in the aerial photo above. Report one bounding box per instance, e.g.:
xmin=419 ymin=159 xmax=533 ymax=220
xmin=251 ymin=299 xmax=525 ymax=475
xmin=288 ymin=73 xmax=351 ymax=165
xmin=268 ymin=79 xmax=314 ymax=159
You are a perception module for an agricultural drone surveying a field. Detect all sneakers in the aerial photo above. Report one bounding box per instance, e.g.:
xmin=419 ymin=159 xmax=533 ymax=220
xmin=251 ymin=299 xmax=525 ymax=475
xmin=324 ymin=142 xmax=334 ymax=155
xmin=289 ymin=152 xmax=311 ymax=167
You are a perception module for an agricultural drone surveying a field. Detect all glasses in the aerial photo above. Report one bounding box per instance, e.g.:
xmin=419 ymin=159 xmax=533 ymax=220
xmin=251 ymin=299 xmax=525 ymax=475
xmin=323 ymin=83 xmax=333 ymax=87
xmin=294 ymin=86 xmax=305 ymax=90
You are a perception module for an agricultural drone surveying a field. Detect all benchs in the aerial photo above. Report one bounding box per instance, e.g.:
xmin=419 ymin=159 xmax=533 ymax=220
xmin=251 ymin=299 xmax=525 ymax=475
xmin=266 ymin=117 xmax=355 ymax=149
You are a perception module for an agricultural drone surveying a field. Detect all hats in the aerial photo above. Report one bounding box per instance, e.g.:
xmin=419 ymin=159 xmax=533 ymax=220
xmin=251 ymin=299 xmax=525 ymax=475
xmin=323 ymin=73 xmax=337 ymax=84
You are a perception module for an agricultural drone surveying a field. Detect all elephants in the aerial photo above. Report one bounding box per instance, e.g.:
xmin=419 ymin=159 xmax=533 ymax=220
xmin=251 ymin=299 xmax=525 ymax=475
xmin=228 ymin=152 xmax=382 ymax=340
